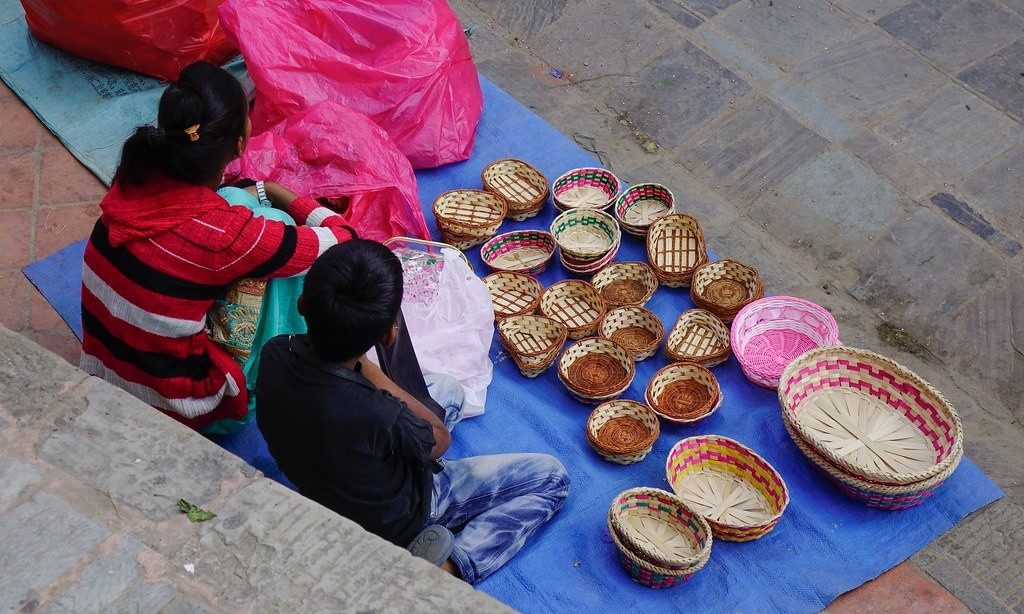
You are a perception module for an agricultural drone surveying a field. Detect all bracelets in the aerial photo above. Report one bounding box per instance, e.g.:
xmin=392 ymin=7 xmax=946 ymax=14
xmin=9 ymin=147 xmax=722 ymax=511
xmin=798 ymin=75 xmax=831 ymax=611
xmin=354 ymin=361 xmax=362 ymax=372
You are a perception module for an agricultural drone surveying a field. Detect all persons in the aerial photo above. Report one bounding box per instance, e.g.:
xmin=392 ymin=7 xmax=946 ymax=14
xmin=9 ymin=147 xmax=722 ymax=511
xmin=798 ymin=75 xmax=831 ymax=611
xmin=79 ymin=61 xmax=359 ymax=435
xmin=255 ymin=238 xmax=571 ymax=587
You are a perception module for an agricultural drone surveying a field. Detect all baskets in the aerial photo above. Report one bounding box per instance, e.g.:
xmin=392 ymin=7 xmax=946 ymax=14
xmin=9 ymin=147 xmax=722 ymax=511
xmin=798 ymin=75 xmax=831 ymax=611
xmin=384 ymin=154 xmax=964 ymax=588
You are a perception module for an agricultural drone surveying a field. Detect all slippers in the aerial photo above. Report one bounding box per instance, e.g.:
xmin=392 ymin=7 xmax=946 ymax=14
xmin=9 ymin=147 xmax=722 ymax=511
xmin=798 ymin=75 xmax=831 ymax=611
xmin=407 ymin=524 xmax=456 ymax=568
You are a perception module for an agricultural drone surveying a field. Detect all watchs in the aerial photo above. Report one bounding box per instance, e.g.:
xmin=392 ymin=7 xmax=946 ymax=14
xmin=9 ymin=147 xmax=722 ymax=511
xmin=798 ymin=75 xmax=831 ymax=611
xmin=256 ymin=180 xmax=272 ymax=208
